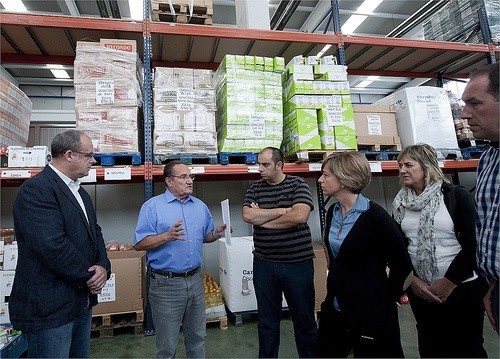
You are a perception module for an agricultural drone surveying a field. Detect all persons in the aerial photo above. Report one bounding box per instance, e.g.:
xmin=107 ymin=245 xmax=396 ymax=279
xmin=459 ymin=61 xmax=500 ymax=337
xmin=242 ymin=146 xmax=320 ymax=359
xmin=131 ymin=161 xmax=233 ymax=359
xmin=390 ymin=144 xmax=488 ymax=359
xmin=9 ymin=129 xmax=112 ymax=359
xmin=318 ymin=150 xmax=412 ymax=359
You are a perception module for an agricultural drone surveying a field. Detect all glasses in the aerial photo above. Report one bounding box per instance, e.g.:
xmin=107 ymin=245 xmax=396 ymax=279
xmin=173 ymin=174 xmax=196 ymax=180
xmin=72 ymin=149 xmax=94 ymax=159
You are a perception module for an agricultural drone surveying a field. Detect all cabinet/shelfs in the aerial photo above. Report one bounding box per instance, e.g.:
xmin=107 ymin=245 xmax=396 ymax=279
xmin=0 ymin=0 xmax=500 ymax=339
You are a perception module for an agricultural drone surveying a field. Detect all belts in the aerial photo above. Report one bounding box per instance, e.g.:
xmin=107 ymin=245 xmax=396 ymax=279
xmin=152 ymin=268 xmax=199 ymax=277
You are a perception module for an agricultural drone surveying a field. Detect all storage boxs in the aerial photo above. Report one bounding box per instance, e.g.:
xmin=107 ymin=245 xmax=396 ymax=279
xmin=0 ymin=0 xmax=500 ymax=359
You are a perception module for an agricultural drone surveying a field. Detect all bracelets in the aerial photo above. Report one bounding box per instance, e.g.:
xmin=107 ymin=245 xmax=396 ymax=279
xmin=212 ymin=233 xmax=217 ymax=240
